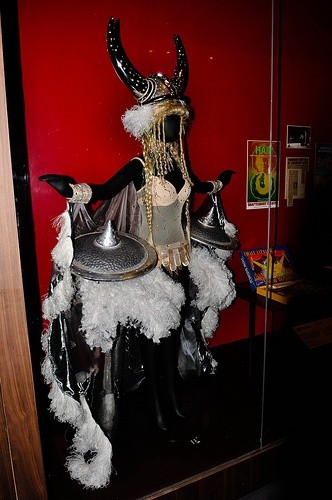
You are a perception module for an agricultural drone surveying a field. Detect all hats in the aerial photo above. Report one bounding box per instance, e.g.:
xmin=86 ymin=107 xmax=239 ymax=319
xmin=104 ymin=14 xmax=192 ymax=135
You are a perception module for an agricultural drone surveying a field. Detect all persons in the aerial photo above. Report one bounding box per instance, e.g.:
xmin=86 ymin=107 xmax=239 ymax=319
xmin=38 ymin=81 xmax=234 ymax=447
